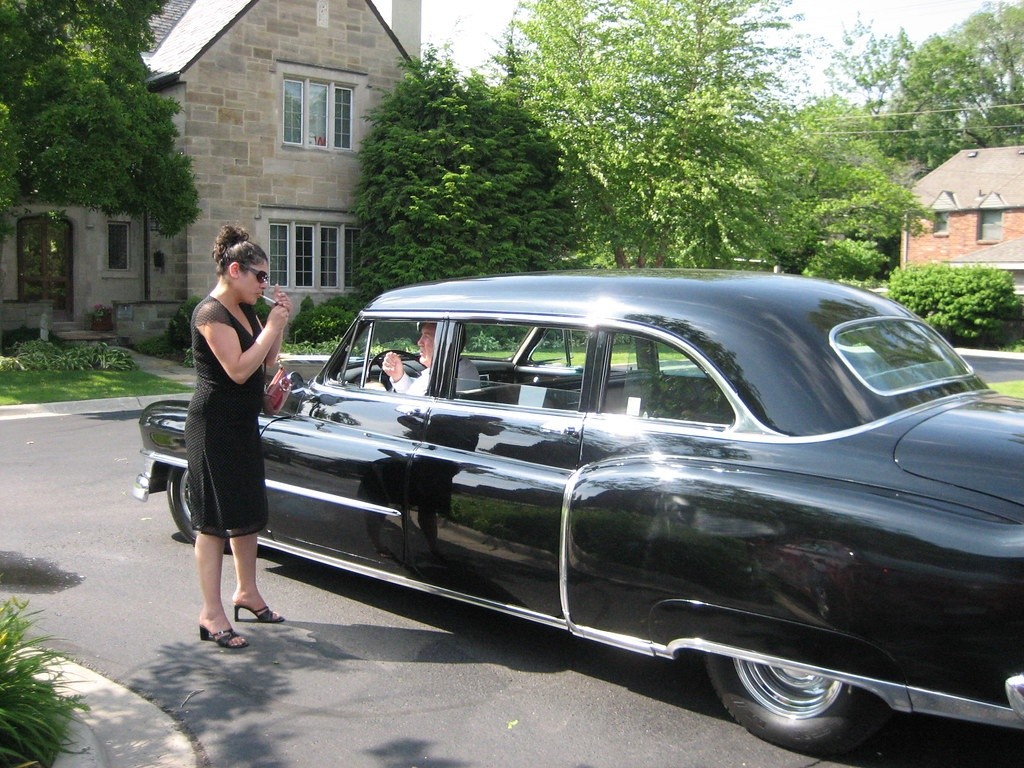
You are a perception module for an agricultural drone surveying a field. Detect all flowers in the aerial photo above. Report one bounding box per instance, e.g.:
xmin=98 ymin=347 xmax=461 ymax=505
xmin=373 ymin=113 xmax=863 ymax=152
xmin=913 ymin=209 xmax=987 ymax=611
xmin=94 ymin=304 xmax=113 ymax=323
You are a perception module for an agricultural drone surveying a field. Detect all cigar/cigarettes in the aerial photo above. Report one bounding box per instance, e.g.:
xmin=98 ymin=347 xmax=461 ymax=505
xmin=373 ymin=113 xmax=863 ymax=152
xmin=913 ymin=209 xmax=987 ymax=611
xmin=258 ymin=294 xmax=276 ymax=304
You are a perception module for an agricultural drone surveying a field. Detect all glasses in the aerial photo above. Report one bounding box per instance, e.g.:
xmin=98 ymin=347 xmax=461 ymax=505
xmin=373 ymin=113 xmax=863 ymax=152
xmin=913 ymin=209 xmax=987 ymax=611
xmin=240 ymin=262 xmax=269 ymax=284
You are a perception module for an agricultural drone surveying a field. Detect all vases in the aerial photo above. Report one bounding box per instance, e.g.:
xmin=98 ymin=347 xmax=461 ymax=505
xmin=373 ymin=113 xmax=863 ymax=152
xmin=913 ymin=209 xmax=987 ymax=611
xmin=91 ymin=321 xmax=113 ymax=332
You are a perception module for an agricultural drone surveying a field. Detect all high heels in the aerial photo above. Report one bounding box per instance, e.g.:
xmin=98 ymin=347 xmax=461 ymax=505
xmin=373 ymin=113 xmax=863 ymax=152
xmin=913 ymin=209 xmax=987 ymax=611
xmin=199 ymin=624 xmax=249 ymax=648
xmin=234 ymin=603 xmax=285 ymax=623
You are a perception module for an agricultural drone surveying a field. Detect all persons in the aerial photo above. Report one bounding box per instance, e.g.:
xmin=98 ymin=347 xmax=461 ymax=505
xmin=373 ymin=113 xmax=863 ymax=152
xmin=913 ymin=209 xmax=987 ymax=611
xmin=383 ymin=321 xmax=479 ymax=400
xmin=360 ymin=416 xmax=504 ymax=560
xmin=184 ymin=224 xmax=294 ymax=649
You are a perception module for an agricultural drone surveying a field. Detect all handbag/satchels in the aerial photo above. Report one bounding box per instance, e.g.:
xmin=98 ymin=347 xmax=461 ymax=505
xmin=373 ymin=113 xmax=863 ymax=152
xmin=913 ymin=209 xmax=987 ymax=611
xmin=262 ymin=364 xmax=292 ymax=416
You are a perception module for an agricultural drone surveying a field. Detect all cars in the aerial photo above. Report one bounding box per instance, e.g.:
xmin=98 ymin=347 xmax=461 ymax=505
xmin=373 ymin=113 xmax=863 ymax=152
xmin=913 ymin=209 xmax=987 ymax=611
xmin=129 ymin=270 xmax=1023 ymax=755
